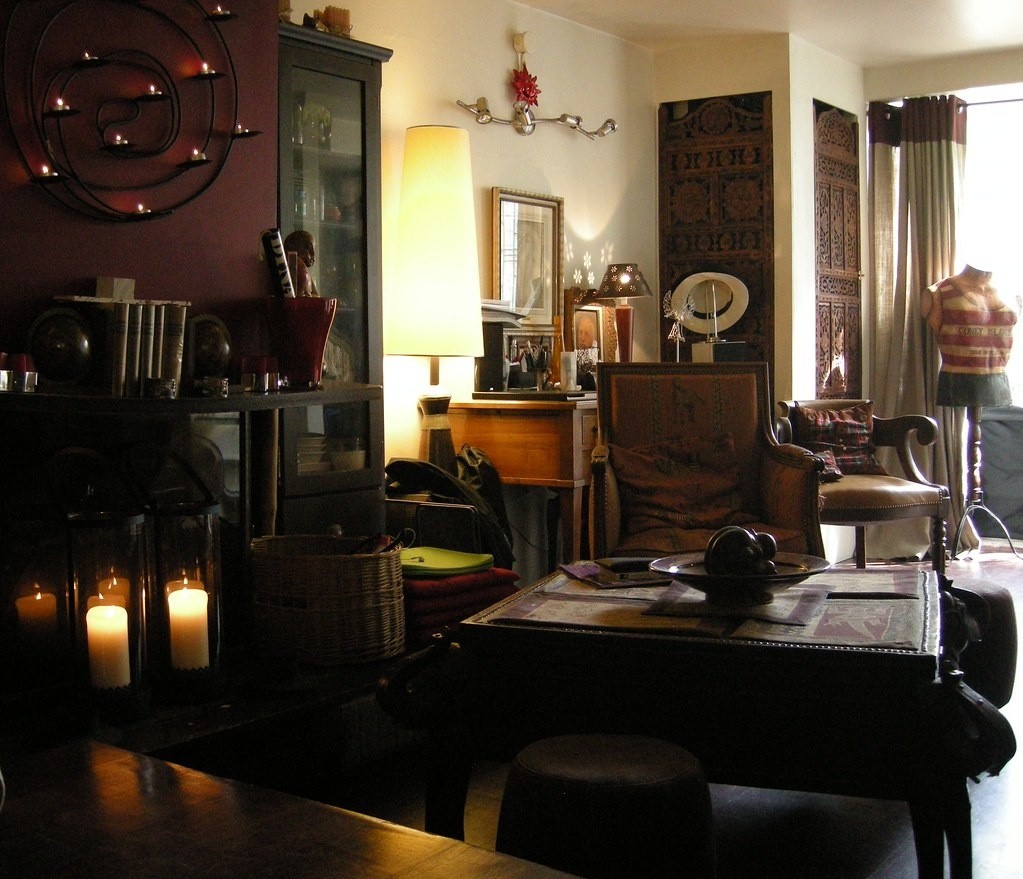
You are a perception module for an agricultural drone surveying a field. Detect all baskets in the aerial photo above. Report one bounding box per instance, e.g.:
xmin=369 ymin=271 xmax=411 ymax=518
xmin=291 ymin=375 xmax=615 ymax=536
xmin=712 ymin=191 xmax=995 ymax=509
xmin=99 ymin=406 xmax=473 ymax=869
xmin=247 ymin=533 xmax=406 ymax=667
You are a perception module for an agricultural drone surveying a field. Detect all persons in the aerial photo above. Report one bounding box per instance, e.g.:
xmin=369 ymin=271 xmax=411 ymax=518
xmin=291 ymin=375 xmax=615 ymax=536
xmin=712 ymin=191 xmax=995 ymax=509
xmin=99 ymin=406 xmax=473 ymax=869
xmin=578 ymin=317 xmax=595 ymax=348
xmin=520 ymin=340 xmax=552 ymax=374
xmin=921 ymin=261 xmax=1017 ymax=408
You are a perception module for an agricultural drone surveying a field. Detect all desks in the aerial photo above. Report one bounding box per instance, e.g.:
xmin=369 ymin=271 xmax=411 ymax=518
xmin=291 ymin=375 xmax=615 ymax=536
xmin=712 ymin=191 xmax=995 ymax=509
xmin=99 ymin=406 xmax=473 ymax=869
xmin=418 ymin=398 xmax=601 ymax=565
xmin=0 ymin=739 xmax=583 ymax=879
xmin=381 ymin=556 xmax=1017 ymax=879
xmin=0 ymin=380 xmax=380 ymax=538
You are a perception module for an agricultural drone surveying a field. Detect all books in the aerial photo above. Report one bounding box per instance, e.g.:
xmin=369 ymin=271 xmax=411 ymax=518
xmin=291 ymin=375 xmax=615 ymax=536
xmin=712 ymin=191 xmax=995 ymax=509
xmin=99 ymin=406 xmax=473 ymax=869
xmin=61 ymin=300 xmax=188 ymax=396
xmin=595 ymin=556 xmax=679 ymax=581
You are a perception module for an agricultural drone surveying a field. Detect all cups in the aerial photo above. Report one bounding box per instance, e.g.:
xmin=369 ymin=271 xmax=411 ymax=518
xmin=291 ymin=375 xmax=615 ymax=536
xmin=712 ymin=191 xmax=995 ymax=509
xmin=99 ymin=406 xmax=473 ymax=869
xmin=560 ymin=352 xmax=577 ymax=390
xmin=295 ymin=432 xmax=330 ymax=472
xmin=202 ymin=376 xmax=229 ymax=398
xmin=241 ymin=357 xmax=269 ymax=371
xmin=527 ymin=368 xmax=552 ymax=390
xmin=142 ymin=378 xmax=176 ymax=399
xmin=330 ymin=451 xmax=366 ymax=471
xmin=241 ymin=371 xmax=279 ymax=391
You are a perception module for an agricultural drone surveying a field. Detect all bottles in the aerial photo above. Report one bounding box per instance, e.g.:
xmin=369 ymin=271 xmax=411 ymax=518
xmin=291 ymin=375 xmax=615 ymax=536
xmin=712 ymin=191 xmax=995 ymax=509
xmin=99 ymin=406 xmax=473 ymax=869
xmin=352 ymin=437 xmax=360 ymax=451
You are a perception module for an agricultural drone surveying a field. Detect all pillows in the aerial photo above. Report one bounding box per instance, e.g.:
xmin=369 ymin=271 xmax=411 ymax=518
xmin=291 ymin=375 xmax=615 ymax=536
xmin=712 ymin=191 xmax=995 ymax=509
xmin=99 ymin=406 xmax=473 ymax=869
xmin=607 ymin=432 xmax=758 ymax=540
xmin=794 ymin=400 xmax=890 ymax=476
xmin=815 ymin=448 xmax=843 ymax=483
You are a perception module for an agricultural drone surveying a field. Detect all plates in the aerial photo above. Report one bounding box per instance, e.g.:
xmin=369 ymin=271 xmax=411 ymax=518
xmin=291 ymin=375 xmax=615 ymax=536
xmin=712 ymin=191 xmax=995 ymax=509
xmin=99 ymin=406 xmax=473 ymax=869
xmin=648 ymin=551 xmax=830 ymax=606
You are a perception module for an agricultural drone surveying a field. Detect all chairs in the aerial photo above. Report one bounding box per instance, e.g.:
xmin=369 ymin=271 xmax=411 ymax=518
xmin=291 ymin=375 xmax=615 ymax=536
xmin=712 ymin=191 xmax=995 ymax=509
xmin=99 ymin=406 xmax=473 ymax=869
xmin=588 ymin=362 xmax=826 ymax=559
xmin=777 ymin=398 xmax=953 ymax=575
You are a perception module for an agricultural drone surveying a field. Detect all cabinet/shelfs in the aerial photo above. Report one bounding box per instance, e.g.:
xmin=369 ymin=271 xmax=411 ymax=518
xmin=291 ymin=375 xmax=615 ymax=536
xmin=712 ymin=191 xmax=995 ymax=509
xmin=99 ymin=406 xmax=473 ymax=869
xmin=278 ymin=20 xmax=393 ymax=497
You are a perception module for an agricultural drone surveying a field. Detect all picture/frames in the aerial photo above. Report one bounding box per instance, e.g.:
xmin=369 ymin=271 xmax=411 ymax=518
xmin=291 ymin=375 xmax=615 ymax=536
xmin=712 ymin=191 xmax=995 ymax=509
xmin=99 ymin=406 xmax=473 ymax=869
xmin=503 ymin=330 xmax=555 ymax=390
xmin=493 ymin=186 xmax=564 ymax=329
xmin=563 ymin=287 xmax=618 ymax=372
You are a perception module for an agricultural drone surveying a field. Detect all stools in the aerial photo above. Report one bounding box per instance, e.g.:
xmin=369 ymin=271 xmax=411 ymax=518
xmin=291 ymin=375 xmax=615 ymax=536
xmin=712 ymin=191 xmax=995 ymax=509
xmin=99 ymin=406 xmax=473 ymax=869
xmin=496 ymin=734 xmax=717 ymax=879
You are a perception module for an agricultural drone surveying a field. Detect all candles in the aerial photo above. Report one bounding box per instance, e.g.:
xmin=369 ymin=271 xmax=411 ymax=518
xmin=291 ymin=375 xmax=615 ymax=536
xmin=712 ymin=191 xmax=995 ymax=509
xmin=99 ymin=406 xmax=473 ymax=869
xmin=136 ymin=202 xmax=152 ymax=214
xmin=192 ymin=148 xmax=207 ymax=161
xmin=201 ymin=61 xmax=215 ymax=74
xmin=84 ymin=605 xmax=129 ymax=690
xmin=235 ymin=124 xmax=249 ymax=135
xmin=167 ymin=588 xmax=210 ymax=670
xmin=211 ymin=5 xmax=230 ymax=15
xmin=148 ymin=84 xmax=163 ymax=95
xmin=114 ymin=134 xmax=128 ymax=145
xmin=87 ymin=592 xmax=127 ymax=614
xmin=51 ymin=98 xmax=70 ymax=111
xmin=83 ymin=53 xmax=99 ymax=60
xmin=165 ymin=576 xmax=205 ymax=618
xmin=41 ymin=164 xmax=58 ymax=176
xmin=99 ymin=576 xmax=131 ymax=606
xmin=16 ymin=591 xmax=58 ymax=636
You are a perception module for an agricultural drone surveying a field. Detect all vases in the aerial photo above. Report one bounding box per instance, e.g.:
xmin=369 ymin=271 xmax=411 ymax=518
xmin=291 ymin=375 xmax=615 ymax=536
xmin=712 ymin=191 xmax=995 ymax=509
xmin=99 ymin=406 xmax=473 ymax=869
xmin=263 ymin=294 xmax=340 ymax=385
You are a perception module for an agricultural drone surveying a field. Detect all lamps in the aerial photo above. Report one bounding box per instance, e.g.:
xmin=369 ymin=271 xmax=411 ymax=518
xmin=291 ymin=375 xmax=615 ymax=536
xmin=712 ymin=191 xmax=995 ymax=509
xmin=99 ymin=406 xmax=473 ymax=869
xmin=383 ymin=125 xmax=483 ymax=501
xmin=595 ymin=263 xmax=654 ymax=362
xmin=456 ymin=30 xmax=619 ymax=141
